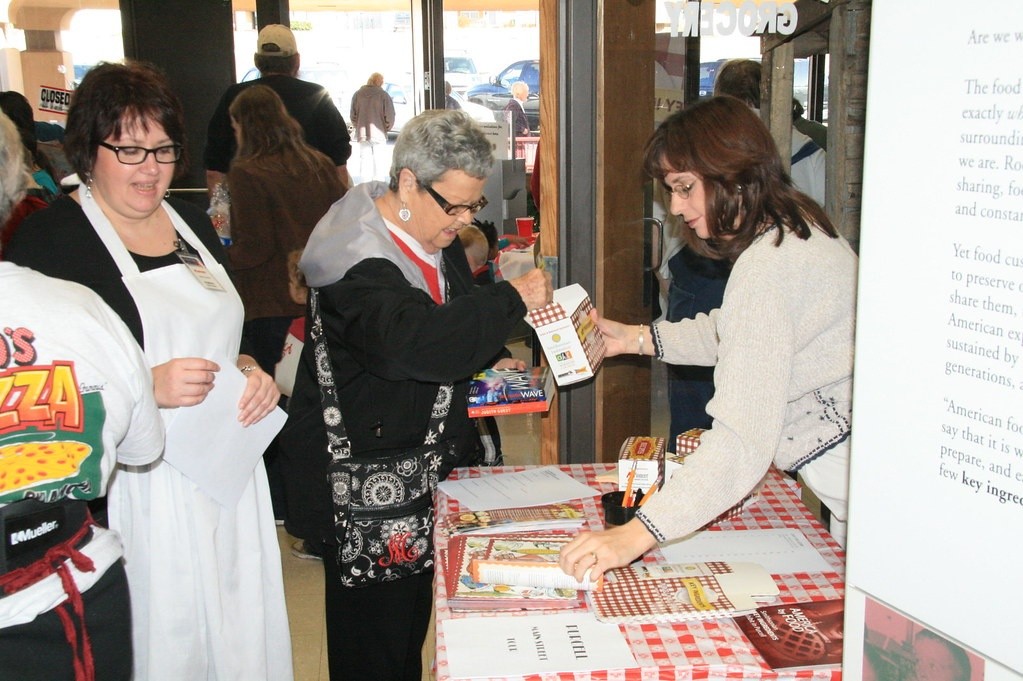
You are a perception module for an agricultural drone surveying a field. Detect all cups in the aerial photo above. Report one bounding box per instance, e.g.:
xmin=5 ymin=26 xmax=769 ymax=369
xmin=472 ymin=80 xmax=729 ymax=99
xmin=516 ymin=218 xmax=534 ymax=238
xmin=601 ymin=492 xmax=644 ymax=563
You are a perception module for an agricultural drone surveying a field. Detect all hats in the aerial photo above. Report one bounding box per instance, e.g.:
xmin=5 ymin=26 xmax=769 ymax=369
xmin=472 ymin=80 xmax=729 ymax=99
xmin=257 ymin=24 xmax=298 ymax=57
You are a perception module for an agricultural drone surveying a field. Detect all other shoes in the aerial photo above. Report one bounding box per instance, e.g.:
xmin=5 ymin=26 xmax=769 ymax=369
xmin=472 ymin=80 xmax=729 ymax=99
xmin=275 ymin=499 xmax=288 ymax=525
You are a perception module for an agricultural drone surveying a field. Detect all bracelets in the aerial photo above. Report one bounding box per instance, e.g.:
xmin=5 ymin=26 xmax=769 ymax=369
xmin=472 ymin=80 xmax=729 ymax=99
xmin=639 ymin=324 xmax=645 ymax=355
xmin=240 ymin=365 xmax=257 ymax=372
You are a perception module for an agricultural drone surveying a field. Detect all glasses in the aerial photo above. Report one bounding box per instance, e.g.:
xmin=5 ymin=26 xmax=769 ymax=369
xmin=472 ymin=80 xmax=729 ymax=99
xmin=100 ymin=141 xmax=185 ymax=165
xmin=667 ymin=177 xmax=700 ymax=199
xmin=426 ymin=186 xmax=491 ymax=217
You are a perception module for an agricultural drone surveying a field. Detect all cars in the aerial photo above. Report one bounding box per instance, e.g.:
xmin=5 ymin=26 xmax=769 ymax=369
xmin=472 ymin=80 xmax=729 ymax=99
xmin=238 ymin=65 xmax=261 ymax=83
xmin=379 ymin=73 xmax=494 ymax=136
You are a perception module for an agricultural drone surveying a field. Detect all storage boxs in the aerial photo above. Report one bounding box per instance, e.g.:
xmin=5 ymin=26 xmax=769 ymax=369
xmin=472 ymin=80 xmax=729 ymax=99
xmin=618 ymin=426 xmax=765 ymax=509
xmin=528 ymin=283 xmax=611 ymax=387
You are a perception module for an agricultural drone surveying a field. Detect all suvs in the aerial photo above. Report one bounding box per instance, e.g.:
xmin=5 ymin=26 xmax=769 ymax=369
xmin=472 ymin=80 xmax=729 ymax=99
xmin=442 ymin=50 xmax=479 ymax=96
xmin=698 ymin=57 xmax=828 ymax=114
xmin=467 ymin=58 xmax=540 ymax=126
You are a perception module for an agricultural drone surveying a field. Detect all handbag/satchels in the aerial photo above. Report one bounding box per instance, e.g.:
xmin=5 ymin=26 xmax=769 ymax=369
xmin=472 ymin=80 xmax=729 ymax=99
xmin=330 ymin=447 xmax=437 ymax=588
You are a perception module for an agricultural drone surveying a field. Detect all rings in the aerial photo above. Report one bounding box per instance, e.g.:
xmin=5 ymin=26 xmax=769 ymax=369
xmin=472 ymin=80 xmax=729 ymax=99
xmin=589 ymin=552 xmax=600 ymax=564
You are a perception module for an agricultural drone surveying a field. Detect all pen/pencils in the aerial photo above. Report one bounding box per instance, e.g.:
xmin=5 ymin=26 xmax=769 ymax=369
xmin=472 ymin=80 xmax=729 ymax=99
xmin=626 ymin=489 xmax=634 ymax=508
xmin=622 ymin=460 xmax=637 ymax=507
xmin=635 ymin=488 xmax=642 ymax=507
xmin=630 ymin=494 xmax=636 ymax=507
xmin=638 ymin=475 xmax=663 ymax=505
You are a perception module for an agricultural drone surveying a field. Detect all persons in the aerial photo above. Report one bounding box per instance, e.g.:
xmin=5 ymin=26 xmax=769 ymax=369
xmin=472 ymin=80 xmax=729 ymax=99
xmin=1 ymin=91 xmax=60 ymax=265
xmin=445 ymin=58 xmax=827 ymax=454
xmin=0 ymin=61 xmax=294 ymax=681
xmin=559 ymin=93 xmax=858 ymax=583
xmin=0 ymin=260 xmax=166 ymax=681
xmin=203 ymin=23 xmax=357 ymax=558
xmin=913 ymin=628 xmax=972 ymax=681
xmin=283 ymin=110 xmax=554 ymax=681
xmin=350 ymin=73 xmax=395 ymax=183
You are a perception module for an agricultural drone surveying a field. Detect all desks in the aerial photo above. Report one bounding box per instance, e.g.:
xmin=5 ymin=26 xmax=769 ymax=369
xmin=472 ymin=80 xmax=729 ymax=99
xmin=428 ymin=441 xmax=846 ymax=681
xmin=496 ymin=234 xmax=541 ymax=282
